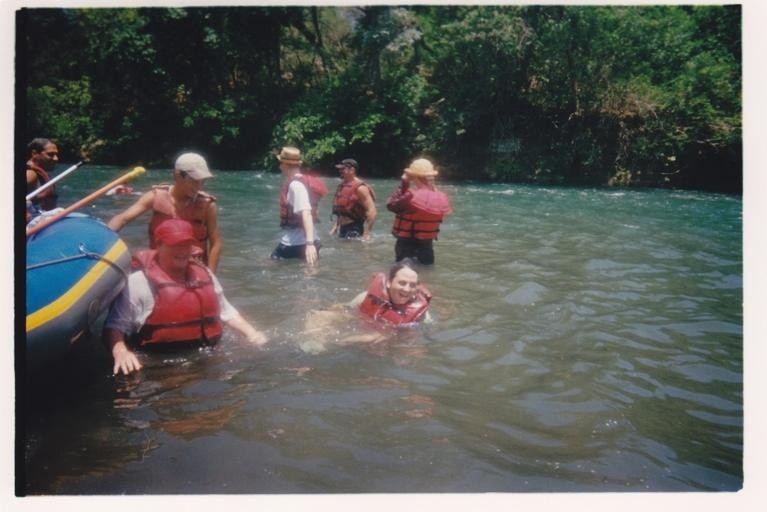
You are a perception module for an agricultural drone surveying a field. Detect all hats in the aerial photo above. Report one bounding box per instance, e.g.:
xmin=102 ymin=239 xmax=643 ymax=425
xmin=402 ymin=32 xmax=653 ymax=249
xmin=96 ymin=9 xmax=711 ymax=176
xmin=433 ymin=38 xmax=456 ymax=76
xmin=404 ymin=158 xmax=437 ymax=178
xmin=155 ymin=218 xmax=197 ymax=244
xmin=176 ymin=152 xmax=213 ymax=181
xmin=276 ymin=147 xmax=302 ymax=165
xmin=336 ymin=158 xmax=358 ymax=169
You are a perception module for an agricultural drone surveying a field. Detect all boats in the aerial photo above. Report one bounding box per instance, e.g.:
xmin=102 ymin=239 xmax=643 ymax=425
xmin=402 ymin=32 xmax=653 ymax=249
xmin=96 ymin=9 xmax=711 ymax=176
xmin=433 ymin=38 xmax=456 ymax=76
xmin=26 ymin=207 xmax=134 ymax=380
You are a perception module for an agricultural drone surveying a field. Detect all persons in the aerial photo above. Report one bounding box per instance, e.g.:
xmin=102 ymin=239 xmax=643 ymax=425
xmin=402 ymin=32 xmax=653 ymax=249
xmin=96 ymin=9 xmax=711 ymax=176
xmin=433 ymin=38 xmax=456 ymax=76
xmin=329 ymin=159 xmax=377 ymax=242
xmin=106 ymin=219 xmax=256 ymax=374
xmin=351 ymin=258 xmax=432 ymax=328
xmin=106 ymin=153 xmax=222 ymax=274
xmin=386 ymin=158 xmax=451 ymax=267
xmin=271 ymin=147 xmax=327 ymax=264
xmin=25 ymin=136 xmax=59 ymax=220
